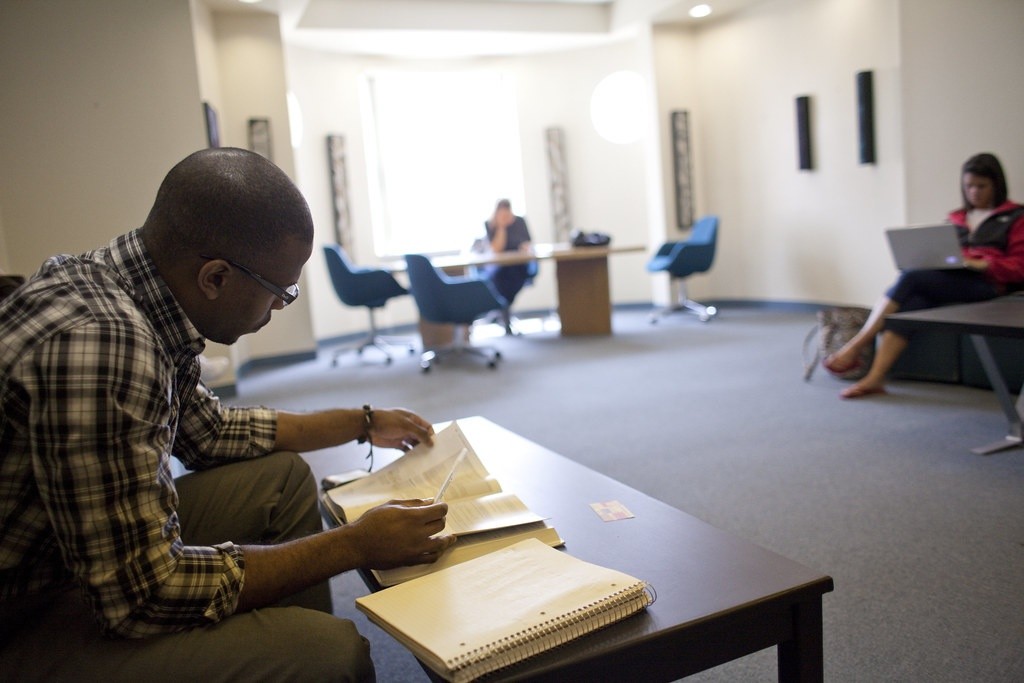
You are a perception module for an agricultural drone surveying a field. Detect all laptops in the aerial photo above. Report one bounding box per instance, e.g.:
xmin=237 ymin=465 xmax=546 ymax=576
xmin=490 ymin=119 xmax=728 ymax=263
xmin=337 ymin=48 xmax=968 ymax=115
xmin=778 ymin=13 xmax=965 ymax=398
xmin=886 ymin=223 xmax=976 ymax=272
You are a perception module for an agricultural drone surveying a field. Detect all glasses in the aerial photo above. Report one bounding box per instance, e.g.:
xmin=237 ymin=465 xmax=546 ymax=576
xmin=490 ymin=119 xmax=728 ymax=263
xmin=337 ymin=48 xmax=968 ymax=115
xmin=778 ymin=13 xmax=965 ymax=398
xmin=201 ymin=253 xmax=299 ymax=307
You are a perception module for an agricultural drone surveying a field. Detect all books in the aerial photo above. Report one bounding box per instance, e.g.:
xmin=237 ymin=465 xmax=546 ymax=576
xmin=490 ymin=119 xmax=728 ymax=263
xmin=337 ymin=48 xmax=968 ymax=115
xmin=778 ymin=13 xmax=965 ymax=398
xmin=355 ymin=538 xmax=657 ymax=683
xmin=322 ymin=423 xmax=565 ymax=589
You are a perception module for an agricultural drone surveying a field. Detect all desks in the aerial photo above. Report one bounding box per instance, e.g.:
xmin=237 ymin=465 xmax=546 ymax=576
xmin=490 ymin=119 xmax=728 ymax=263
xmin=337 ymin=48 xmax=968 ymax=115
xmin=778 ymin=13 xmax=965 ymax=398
xmin=309 ymin=415 xmax=833 ymax=683
xmin=380 ymin=242 xmax=647 ymax=348
xmin=886 ymin=299 xmax=1024 ymax=456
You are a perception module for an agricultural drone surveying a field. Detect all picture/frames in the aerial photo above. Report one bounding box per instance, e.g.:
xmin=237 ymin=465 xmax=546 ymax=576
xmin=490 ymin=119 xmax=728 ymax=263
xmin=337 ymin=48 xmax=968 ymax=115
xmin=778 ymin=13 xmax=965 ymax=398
xmin=326 ymin=134 xmax=352 ymax=246
xmin=672 ymin=111 xmax=696 ymax=227
xmin=205 ymin=103 xmax=219 ymax=146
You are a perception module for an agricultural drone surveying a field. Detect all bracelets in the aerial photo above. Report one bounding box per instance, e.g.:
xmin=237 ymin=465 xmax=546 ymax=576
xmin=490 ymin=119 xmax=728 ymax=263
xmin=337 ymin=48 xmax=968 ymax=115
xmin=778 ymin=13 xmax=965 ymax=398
xmin=358 ymin=405 xmax=373 ymax=472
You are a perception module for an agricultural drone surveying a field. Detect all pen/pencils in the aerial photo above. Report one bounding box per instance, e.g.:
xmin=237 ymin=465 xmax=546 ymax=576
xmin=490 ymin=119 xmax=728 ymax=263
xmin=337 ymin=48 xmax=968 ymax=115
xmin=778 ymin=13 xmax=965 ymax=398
xmin=434 ymin=446 xmax=469 ymax=504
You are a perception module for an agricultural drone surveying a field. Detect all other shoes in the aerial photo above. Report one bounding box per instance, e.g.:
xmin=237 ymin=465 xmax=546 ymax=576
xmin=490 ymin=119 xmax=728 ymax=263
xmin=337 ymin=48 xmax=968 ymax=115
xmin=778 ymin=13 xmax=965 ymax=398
xmin=825 ymin=352 xmax=862 ymax=372
xmin=841 ymin=383 xmax=879 ymax=397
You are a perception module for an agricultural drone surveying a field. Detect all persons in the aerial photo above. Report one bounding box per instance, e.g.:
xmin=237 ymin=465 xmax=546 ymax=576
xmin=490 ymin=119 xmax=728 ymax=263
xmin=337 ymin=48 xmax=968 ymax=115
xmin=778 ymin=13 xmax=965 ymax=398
xmin=823 ymin=153 xmax=1024 ymax=399
xmin=486 ymin=199 xmax=531 ymax=336
xmin=0 ymin=147 xmax=458 ymax=683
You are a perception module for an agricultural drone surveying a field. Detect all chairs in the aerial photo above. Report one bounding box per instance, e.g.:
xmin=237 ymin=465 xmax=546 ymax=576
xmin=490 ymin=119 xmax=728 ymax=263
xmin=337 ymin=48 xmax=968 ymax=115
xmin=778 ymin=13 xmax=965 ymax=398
xmin=322 ymin=245 xmax=415 ymax=365
xmin=405 ymin=250 xmax=505 ymax=371
xmin=643 ymin=215 xmax=722 ymax=323
